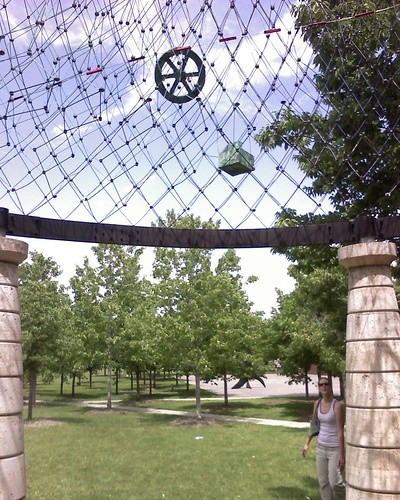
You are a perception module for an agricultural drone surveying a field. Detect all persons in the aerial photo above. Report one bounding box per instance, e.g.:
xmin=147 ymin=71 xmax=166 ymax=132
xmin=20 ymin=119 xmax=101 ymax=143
xmin=273 ymin=358 xmax=282 ymax=376
xmin=302 ymin=377 xmax=347 ymax=500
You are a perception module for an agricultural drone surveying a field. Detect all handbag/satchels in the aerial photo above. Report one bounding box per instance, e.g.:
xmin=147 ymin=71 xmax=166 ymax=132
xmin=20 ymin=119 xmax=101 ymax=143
xmin=309 ymin=419 xmax=320 ymax=436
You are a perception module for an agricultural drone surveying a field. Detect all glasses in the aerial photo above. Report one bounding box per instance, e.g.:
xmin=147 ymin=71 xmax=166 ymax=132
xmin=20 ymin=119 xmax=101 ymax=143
xmin=318 ymin=383 xmax=331 ymax=386
